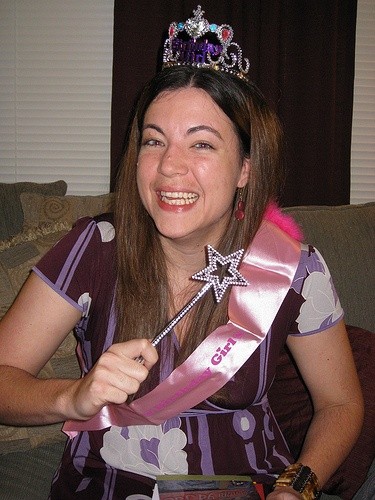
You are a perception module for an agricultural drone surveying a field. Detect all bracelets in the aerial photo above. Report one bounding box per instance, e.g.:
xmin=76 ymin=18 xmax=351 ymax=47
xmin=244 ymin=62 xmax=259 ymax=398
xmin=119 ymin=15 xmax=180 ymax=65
xmin=0 ymin=67 xmax=364 ymax=500
xmin=273 ymin=463 xmax=321 ymax=500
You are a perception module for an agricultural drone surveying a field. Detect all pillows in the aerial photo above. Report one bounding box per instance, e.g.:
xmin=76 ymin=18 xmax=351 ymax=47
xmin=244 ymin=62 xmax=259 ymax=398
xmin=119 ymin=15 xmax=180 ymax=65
xmin=268 ymin=324 xmax=375 ymax=500
xmin=0 ymin=182 xmax=113 ymax=453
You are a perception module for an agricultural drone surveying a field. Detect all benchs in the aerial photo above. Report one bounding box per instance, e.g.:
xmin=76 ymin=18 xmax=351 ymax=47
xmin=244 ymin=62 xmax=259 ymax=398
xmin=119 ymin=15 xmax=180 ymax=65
xmin=0 ymin=181 xmax=375 ymax=500
